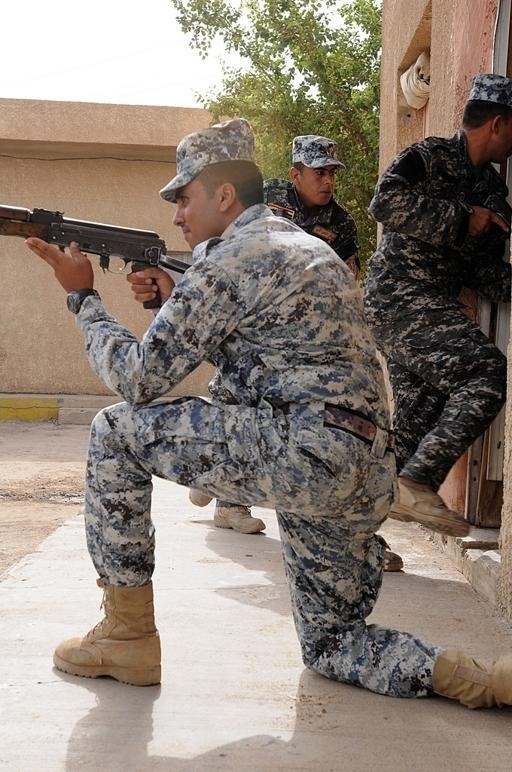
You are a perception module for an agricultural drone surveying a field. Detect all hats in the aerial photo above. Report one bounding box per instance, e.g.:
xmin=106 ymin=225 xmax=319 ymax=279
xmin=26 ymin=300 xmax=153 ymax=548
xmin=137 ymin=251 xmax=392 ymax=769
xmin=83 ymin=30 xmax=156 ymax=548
xmin=293 ymin=135 xmax=346 ymax=170
xmin=159 ymin=118 xmax=256 ymax=203
xmin=468 ymin=74 xmax=512 ymax=106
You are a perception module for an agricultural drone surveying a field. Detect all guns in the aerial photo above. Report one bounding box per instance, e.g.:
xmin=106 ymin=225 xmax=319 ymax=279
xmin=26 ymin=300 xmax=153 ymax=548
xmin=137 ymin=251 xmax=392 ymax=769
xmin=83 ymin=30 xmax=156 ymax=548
xmin=0 ymin=205 xmax=191 ymax=309
xmin=483 ymin=188 xmax=512 ymax=228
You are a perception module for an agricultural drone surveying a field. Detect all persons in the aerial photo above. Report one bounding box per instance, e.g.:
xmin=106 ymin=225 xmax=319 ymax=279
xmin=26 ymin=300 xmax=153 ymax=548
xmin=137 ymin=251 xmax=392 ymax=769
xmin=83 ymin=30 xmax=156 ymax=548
xmin=360 ymin=70 xmax=512 ymax=573
xmin=186 ymin=133 xmax=358 ymax=534
xmin=22 ymin=120 xmax=510 ymax=711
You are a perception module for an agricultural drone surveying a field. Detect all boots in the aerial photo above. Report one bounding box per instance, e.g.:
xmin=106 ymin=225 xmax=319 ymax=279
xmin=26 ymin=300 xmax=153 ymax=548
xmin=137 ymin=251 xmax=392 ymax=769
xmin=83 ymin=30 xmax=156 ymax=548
xmin=190 ymin=489 xmax=213 ymax=508
xmin=390 ymin=476 xmax=469 ymax=538
xmin=53 ymin=581 xmax=161 ymax=685
xmin=374 ymin=534 xmax=404 ymax=570
xmin=215 ymin=506 xmax=266 ymax=533
xmin=432 ymin=649 xmax=512 ymax=710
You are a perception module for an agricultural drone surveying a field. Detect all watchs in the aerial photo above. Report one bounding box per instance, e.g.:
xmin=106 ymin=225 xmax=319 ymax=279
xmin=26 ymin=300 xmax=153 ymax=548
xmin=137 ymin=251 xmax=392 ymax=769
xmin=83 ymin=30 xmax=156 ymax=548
xmin=65 ymin=287 xmax=102 ymax=314
